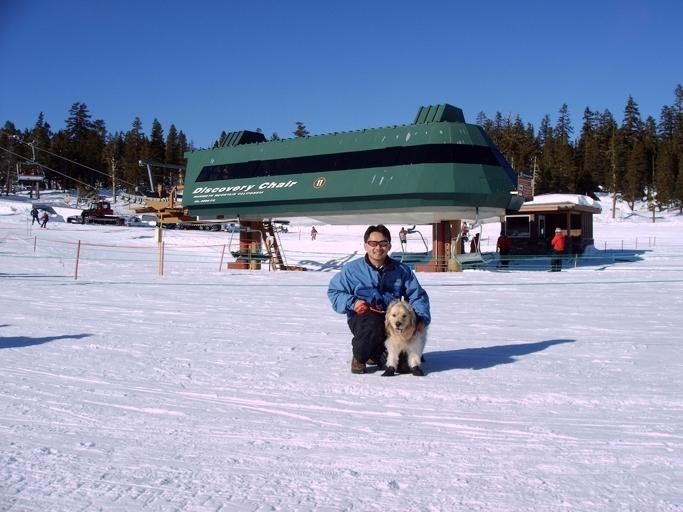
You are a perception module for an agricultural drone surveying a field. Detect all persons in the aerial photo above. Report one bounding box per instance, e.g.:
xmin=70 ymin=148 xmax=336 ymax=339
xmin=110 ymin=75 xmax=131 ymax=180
xmin=461 ymin=221 xmax=469 ymax=242
xmin=470 ymin=233 xmax=480 ymax=252
xmin=29 ymin=207 xmax=42 ymax=226
xmin=39 ymin=212 xmax=49 ymax=228
xmin=310 ymin=225 xmax=318 ymax=241
xmin=398 ymin=227 xmax=408 ymax=244
xmin=545 ymin=226 xmax=565 ymax=272
xmin=495 ymin=230 xmax=511 ymax=268
xmin=326 ymin=223 xmax=432 ymax=376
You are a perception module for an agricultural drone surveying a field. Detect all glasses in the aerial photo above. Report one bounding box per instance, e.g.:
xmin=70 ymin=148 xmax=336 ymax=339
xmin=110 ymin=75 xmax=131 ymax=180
xmin=365 ymin=240 xmax=390 ymax=247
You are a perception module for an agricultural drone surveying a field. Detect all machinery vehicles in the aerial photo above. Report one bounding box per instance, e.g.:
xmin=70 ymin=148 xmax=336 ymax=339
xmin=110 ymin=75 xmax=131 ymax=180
xmin=134 ymin=181 xmax=221 ymax=231
xmin=67 ymin=200 xmax=124 ymax=227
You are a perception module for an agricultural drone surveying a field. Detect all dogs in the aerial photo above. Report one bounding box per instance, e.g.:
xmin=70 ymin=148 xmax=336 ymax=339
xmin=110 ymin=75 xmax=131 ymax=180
xmin=380 ymin=299 xmax=428 ymax=377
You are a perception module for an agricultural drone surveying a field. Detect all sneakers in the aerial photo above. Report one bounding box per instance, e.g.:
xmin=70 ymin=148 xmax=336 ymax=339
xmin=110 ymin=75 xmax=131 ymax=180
xmin=350 ymin=355 xmax=367 ymax=375
